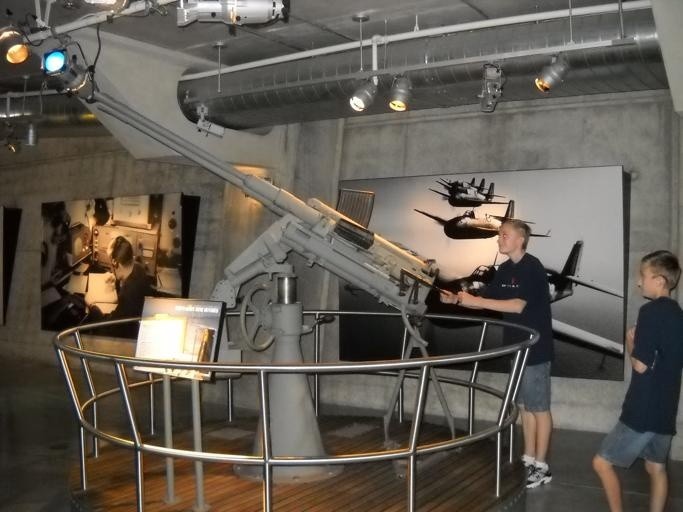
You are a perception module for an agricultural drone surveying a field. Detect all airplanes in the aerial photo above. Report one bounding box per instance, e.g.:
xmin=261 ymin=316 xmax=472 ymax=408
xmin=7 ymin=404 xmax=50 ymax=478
xmin=429 ymin=240 xmax=622 ymax=360
xmin=410 ymin=176 xmax=553 ymax=240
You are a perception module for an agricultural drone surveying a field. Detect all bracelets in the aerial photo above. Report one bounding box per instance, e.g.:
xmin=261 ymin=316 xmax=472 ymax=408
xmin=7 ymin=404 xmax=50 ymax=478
xmin=454 ymin=299 xmax=461 ymax=305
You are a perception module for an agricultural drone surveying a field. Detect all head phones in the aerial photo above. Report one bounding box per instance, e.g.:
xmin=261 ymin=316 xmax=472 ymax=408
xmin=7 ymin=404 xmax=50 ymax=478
xmin=111 ymin=236 xmax=125 ymax=268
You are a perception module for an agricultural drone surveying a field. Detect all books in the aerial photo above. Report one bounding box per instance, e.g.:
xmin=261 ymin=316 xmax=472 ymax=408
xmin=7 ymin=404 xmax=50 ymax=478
xmin=63 ymin=272 xmax=119 ymax=317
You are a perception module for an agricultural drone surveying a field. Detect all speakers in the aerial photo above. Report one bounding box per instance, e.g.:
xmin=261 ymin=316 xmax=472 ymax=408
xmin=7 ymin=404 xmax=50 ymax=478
xmin=69 ymin=224 xmax=90 ymax=265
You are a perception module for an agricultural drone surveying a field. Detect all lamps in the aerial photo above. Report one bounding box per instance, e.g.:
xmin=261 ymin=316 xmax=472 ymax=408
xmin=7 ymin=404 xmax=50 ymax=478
xmin=388 ymin=77 xmax=413 ymax=111
xmin=1 ymin=23 xmax=30 ymax=64
xmin=25 ymin=121 xmax=38 ymax=146
xmin=480 ymin=67 xmax=506 ymax=112
xmin=176 ymin=0 xmax=286 ymax=27
xmin=349 ymin=81 xmax=378 ymax=112
xmin=535 ymin=60 xmax=571 ymax=94
xmin=41 ymin=48 xmax=99 ymax=103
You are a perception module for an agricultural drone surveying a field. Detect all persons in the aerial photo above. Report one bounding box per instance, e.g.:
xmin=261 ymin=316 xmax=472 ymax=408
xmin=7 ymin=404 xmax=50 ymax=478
xmin=437 ymin=218 xmax=552 ymax=489
xmin=591 ymin=251 xmax=682 ymax=512
xmin=87 ymin=234 xmax=153 ymax=337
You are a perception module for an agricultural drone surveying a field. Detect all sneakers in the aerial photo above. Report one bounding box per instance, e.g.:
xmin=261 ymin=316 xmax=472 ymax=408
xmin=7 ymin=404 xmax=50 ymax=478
xmin=521 ymin=461 xmax=551 ymax=490
